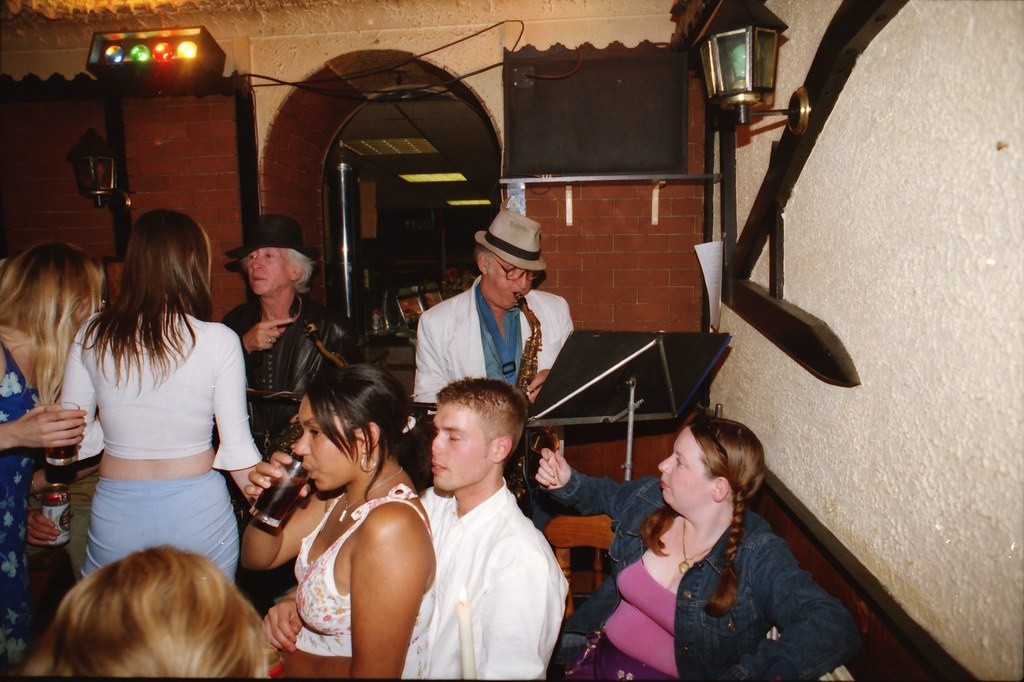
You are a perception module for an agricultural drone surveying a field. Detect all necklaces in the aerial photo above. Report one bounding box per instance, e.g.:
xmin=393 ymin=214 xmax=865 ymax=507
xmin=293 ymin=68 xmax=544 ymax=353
xmin=679 ymin=515 xmax=713 ymax=576
xmin=339 ymin=466 xmax=403 ymax=522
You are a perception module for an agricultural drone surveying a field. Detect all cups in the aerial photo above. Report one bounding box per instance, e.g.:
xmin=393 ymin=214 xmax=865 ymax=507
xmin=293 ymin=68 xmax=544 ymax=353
xmin=249 ymin=459 xmax=313 ymax=528
xmin=44 ymin=401 xmax=81 ymax=467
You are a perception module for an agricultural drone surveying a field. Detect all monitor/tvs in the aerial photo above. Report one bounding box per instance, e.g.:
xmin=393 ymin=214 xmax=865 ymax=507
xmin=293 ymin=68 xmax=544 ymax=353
xmin=504 ymin=50 xmax=690 ymax=178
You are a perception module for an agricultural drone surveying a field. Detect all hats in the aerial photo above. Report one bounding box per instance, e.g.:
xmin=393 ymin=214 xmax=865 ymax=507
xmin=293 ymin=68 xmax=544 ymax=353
xmin=226 ymin=214 xmax=320 ymax=272
xmin=474 ymin=210 xmax=545 ymax=270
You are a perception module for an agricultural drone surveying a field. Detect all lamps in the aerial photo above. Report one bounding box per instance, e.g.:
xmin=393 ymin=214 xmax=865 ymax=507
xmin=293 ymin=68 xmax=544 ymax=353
xmin=691 ymin=1 xmax=812 ymax=139
xmin=86 ymin=25 xmax=226 ymax=88
xmin=62 ymin=126 xmax=131 ymax=215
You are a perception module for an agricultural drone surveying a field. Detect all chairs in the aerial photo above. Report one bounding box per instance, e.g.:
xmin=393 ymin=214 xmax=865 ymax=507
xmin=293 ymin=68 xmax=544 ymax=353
xmin=546 ymin=512 xmax=621 ymax=618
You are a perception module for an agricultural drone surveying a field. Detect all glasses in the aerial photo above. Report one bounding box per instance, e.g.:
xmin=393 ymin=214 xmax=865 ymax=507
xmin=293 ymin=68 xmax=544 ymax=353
xmin=493 ymin=256 xmax=540 ymax=281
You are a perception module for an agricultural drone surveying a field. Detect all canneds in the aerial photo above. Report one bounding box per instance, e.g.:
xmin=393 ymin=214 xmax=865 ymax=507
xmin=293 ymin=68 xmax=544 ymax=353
xmin=40 ymin=483 xmax=72 ymax=549
xmin=267 ymin=650 xmax=284 ymax=679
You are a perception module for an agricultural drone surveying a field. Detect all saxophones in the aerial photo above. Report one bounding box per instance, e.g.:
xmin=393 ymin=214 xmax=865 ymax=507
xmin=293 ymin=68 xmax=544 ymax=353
xmin=507 ymin=292 xmax=559 ymax=509
xmin=263 ymin=318 xmax=347 ymax=465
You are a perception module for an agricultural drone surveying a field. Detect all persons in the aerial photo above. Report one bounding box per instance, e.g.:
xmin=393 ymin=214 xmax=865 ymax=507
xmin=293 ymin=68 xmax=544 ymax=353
xmin=60 ymin=207 xmax=267 ymax=590
xmin=534 ymin=418 xmax=865 ymax=682
xmin=219 ymin=214 xmax=356 ymax=458
xmin=412 ymin=210 xmax=576 ymax=559
xmin=0 ymin=241 xmax=105 ymax=682
xmin=241 ymin=363 xmax=435 ymax=682
xmin=263 ymin=380 xmax=570 ymax=682
xmin=4 ymin=546 xmax=266 ymax=681
